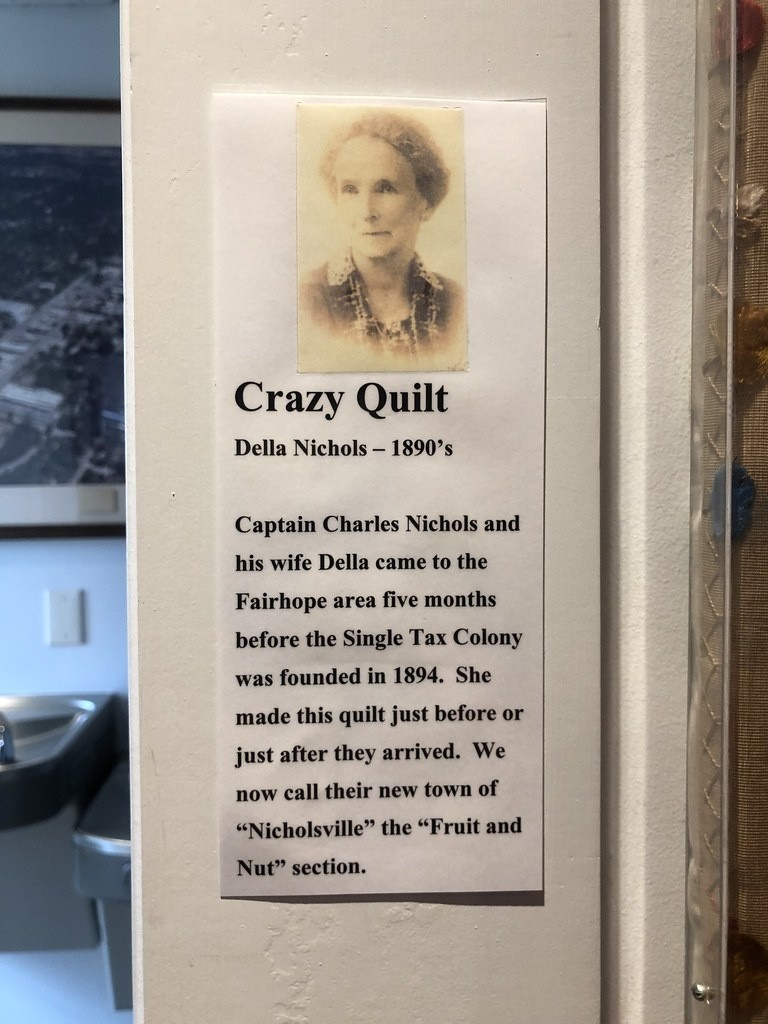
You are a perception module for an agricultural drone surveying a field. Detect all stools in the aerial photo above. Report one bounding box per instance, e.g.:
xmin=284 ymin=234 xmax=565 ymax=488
xmin=70 ymin=754 xmax=134 ymax=1012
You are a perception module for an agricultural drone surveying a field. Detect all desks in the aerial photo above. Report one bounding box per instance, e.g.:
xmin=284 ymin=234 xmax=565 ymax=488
xmin=0 ymin=693 xmax=117 ymax=952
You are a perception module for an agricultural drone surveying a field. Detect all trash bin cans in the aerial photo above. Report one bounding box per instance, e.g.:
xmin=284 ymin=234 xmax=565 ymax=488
xmin=73 ymin=749 xmax=133 ymax=1012
xmin=0 ymin=693 xmax=129 ymax=954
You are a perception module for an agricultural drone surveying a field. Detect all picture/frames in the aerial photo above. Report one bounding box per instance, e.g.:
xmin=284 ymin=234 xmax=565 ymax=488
xmin=0 ymin=91 xmax=124 ymax=540
